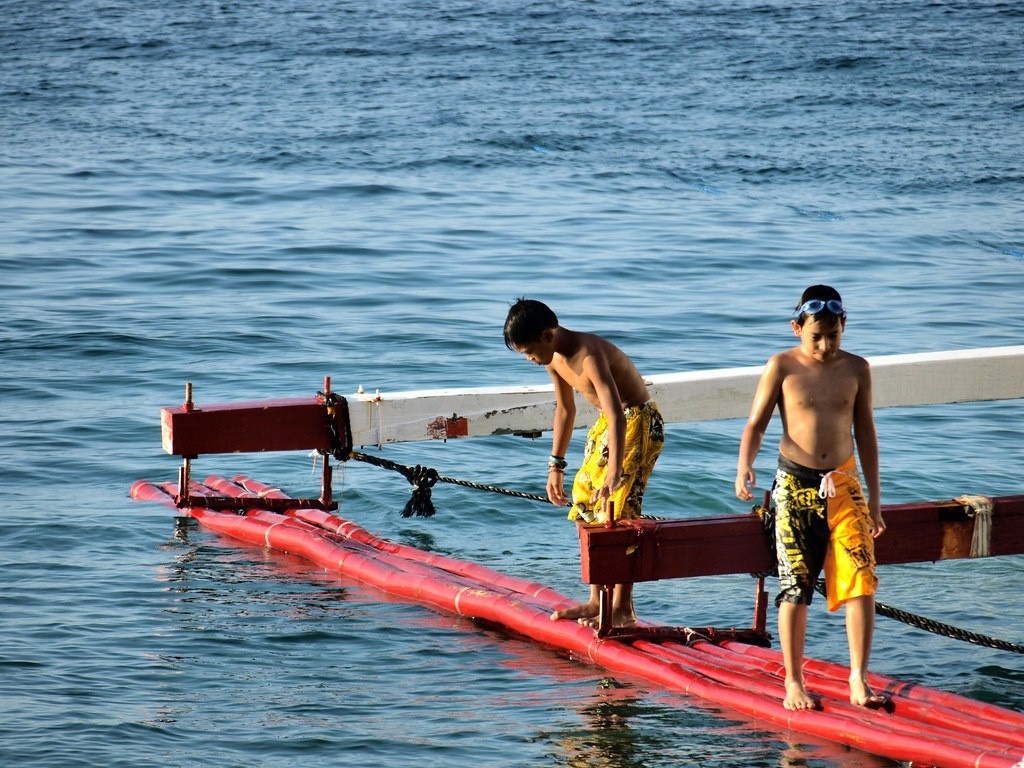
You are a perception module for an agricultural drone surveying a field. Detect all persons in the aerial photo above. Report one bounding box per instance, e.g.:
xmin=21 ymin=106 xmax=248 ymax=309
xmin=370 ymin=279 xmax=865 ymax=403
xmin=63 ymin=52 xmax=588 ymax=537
xmin=502 ymin=300 xmax=664 ymax=630
xmin=733 ymin=285 xmax=886 ymax=710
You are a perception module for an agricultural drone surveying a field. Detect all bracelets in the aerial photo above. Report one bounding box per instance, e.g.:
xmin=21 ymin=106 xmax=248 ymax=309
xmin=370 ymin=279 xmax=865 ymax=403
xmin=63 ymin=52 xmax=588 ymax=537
xmin=547 ymin=455 xmax=567 ymax=473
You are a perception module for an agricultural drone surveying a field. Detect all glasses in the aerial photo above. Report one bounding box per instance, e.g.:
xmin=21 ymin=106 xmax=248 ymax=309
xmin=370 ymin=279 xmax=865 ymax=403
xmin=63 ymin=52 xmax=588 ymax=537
xmin=795 ymin=300 xmax=846 ymax=323
